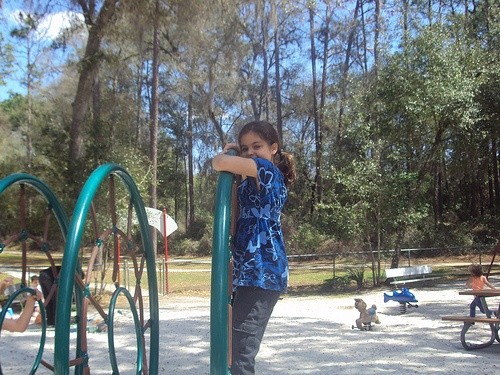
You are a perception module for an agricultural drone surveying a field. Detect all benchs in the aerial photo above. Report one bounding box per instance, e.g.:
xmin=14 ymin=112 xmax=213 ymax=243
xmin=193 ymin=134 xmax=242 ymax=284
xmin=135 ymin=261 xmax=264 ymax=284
xmin=385 ymin=264 xmax=441 ymax=289
xmin=442 ymin=316 xmax=500 ymax=323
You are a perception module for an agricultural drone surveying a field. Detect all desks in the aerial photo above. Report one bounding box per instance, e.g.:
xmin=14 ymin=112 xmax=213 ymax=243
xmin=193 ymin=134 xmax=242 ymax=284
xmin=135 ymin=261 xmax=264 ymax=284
xmin=459 ymin=289 xmax=500 ymax=349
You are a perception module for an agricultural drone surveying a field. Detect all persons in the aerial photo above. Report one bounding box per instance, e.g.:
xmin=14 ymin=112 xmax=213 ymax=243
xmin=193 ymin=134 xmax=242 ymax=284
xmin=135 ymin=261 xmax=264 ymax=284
xmin=466 ymin=264 xmax=498 ymax=326
xmin=39 ymin=266 xmax=86 ymax=328
xmin=212 ymin=120 xmax=297 ymax=375
xmin=28 ymin=275 xmax=45 ymax=312
xmin=2 ymin=288 xmax=43 ymax=332
xmin=0 ymin=277 xmax=16 ymax=312
